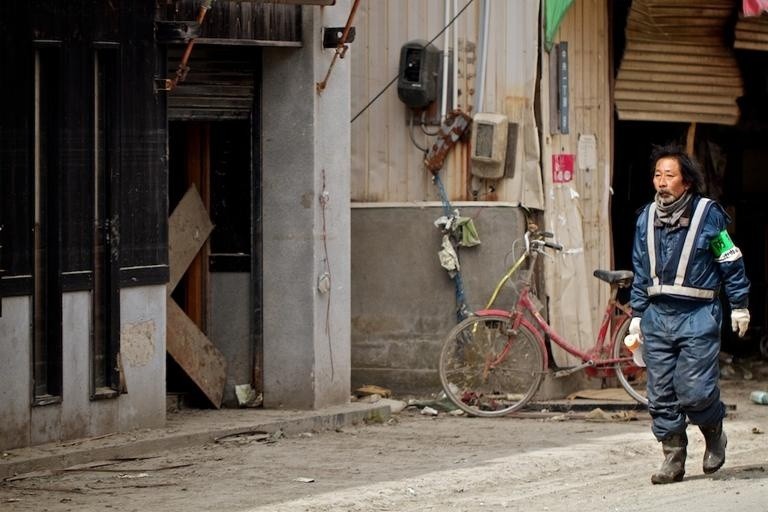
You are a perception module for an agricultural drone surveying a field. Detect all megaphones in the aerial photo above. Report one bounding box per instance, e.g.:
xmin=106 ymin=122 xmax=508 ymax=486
xmin=623 ymin=334 xmax=646 ymax=367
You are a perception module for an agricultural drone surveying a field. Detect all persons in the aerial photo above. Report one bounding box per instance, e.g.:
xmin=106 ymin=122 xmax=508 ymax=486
xmin=628 ymin=146 xmax=751 ymax=483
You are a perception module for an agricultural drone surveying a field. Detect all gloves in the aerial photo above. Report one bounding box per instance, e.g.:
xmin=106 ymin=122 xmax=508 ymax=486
xmin=629 ymin=317 xmax=645 ymax=341
xmin=731 ymin=309 xmax=751 ymax=337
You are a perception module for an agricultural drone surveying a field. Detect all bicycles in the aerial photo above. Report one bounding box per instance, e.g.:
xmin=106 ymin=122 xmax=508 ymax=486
xmin=438 ymin=230 xmax=649 ymax=417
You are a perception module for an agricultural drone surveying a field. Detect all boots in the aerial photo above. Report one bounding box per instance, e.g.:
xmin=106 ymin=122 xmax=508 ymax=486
xmin=698 ymin=418 xmax=727 ymax=474
xmin=652 ymin=432 xmax=688 ymax=484
